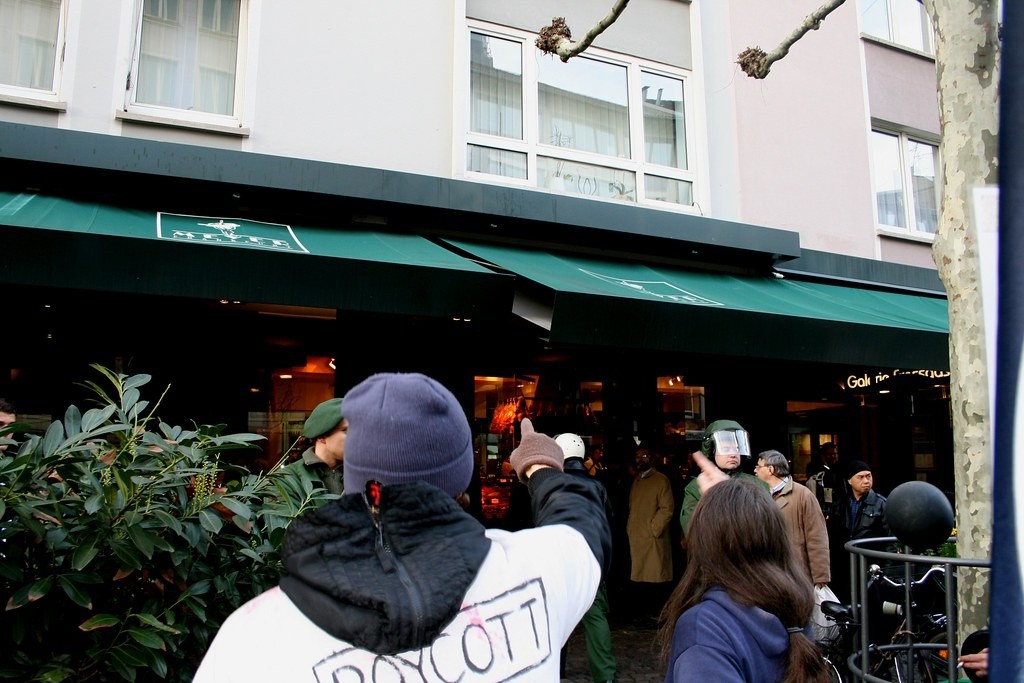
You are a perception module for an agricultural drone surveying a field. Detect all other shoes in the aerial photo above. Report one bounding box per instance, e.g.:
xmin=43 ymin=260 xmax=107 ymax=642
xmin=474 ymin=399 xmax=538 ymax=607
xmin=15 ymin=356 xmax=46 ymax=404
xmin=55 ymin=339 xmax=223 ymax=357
xmin=643 ymin=615 xmax=668 ymax=630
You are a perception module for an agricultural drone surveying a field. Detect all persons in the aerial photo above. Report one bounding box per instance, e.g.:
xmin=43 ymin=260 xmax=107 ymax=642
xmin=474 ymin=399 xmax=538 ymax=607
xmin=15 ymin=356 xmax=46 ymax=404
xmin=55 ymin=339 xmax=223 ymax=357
xmin=190 ymin=371 xmax=610 ymax=683
xmin=263 ymin=398 xmax=350 ymax=540
xmin=502 ymin=419 xmax=957 ymax=683
xmin=956 ymin=615 xmax=989 ymax=683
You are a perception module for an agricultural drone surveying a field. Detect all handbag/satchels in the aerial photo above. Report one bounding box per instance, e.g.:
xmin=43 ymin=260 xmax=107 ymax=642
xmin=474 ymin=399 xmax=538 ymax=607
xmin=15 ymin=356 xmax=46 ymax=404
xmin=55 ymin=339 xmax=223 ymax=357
xmin=805 ymin=475 xmax=817 ymax=496
xmin=810 ymin=584 xmax=841 ymax=628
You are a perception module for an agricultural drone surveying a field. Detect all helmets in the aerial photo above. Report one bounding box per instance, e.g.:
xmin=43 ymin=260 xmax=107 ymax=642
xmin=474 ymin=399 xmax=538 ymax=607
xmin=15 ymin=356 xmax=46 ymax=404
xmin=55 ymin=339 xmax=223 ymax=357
xmin=552 ymin=433 xmax=586 ymax=460
xmin=589 ymin=434 xmax=607 ymax=450
xmin=701 ymin=419 xmax=745 ymax=458
xmin=673 ymin=462 xmax=690 ymax=481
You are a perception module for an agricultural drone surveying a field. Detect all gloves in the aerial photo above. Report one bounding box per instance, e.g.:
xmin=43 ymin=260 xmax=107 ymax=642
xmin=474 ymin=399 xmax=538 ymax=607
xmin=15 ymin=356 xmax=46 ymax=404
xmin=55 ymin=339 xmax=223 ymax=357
xmin=508 ymin=417 xmax=564 ymax=483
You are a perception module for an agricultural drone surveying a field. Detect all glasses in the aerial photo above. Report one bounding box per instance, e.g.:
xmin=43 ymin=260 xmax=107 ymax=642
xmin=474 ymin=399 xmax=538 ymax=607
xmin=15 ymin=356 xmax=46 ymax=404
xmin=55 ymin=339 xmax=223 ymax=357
xmin=635 ymin=454 xmax=648 ymax=458
xmin=755 ymin=465 xmax=765 ymax=470
xmin=681 ymin=468 xmax=688 ymax=471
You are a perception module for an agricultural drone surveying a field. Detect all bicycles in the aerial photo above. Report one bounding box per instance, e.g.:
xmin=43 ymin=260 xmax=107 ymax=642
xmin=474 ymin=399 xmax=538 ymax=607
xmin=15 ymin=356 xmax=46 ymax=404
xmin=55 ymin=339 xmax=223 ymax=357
xmin=816 ymin=564 xmax=958 ymax=683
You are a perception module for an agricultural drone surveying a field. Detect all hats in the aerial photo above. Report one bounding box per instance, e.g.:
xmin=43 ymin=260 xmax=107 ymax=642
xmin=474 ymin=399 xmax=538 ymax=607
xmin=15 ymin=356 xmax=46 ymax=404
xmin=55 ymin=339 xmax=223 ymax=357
xmin=340 ymin=370 xmax=476 ymax=499
xmin=303 ymin=397 xmax=341 ymax=439
xmin=844 ymin=460 xmax=871 ymax=479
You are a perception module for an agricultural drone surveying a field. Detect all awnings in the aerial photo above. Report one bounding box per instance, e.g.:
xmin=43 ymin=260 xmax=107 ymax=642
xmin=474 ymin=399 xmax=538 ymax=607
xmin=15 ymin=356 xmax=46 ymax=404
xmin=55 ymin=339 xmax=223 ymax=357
xmin=0 ymin=172 xmax=497 ymax=371
xmin=441 ymin=237 xmax=949 ymax=333
